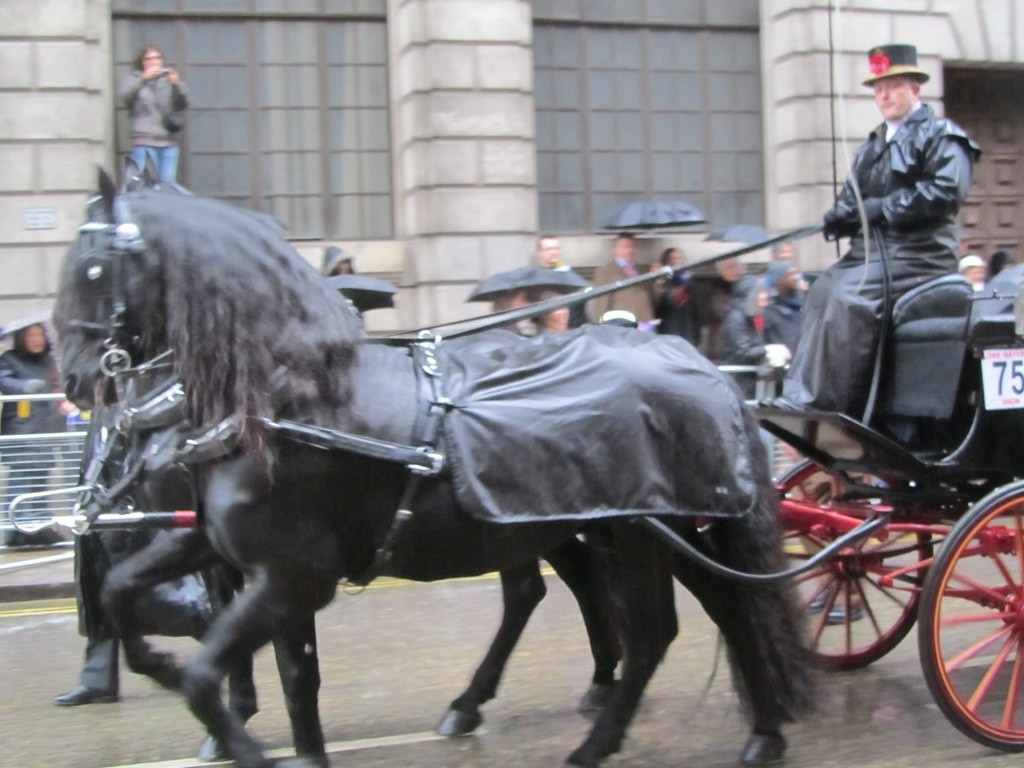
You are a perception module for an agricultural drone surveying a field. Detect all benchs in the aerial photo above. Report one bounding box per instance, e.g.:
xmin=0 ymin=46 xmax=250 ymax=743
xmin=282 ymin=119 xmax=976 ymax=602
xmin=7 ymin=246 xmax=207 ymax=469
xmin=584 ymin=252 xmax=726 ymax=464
xmin=741 ymin=274 xmax=1017 ymax=466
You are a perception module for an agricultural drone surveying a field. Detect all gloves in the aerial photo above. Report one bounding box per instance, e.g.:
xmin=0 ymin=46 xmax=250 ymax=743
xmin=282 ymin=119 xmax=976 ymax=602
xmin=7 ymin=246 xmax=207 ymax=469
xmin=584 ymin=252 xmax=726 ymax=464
xmin=22 ymin=379 xmax=46 ymax=394
xmin=852 ymin=197 xmax=886 ymax=224
xmin=763 ymin=343 xmax=792 ymax=368
xmin=823 ymin=200 xmax=852 ymax=236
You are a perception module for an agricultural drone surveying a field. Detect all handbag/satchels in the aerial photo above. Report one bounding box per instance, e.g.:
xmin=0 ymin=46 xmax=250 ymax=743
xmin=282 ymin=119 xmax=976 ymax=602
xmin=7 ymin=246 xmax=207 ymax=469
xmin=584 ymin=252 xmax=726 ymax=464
xmin=163 ymin=111 xmax=187 ymax=133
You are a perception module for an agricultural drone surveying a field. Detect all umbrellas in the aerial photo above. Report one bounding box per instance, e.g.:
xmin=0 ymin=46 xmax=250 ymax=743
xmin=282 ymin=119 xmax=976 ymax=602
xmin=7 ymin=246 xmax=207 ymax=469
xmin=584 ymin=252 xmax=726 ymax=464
xmin=325 ymin=273 xmax=398 ymax=314
xmin=464 ymin=265 xmax=591 ymax=302
xmin=700 ymin=225 xmax=774 ymax=246
xmin=598 ymin=196 xmax=709 ymax=229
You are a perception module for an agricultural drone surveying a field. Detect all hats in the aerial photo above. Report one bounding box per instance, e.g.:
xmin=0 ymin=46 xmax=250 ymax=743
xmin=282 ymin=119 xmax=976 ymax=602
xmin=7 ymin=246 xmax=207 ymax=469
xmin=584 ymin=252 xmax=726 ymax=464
xmin=958 ymin=255 xmax=987 ymax=272
xmin=862 ymin=44 xmax=929 ymax=87
xmin=766 ymin=260 xmax=791 ymax=287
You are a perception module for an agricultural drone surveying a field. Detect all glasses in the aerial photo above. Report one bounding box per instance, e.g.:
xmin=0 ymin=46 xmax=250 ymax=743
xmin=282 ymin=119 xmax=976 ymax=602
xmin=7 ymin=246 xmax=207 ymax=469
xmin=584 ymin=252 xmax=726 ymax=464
xmin=144 ymin=55 xmax=163 ymax=61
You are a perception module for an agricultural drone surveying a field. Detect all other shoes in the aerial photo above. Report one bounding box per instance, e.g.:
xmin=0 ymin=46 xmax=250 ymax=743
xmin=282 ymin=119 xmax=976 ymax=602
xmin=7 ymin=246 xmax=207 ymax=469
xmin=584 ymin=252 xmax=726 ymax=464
xmin=825 ymin=600 xmax=866 ymax=624
xmin=53 ymin=684 xmax=118 ymax=706
xmin=808 ymin=593 xmax=836 ymax=614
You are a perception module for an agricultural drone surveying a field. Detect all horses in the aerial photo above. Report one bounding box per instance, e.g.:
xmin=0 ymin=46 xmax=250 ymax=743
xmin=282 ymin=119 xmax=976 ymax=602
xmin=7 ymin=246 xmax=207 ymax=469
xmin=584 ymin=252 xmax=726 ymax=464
xmin=51 ymin=149 xmax=830 ymax=768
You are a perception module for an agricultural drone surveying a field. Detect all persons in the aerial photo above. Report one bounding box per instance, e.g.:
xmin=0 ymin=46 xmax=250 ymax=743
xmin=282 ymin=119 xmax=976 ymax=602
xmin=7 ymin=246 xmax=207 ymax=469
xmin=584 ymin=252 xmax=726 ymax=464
xmin=323 ymin=244 xmax=357 ymax=276
xmin=793 ymin=453 xmax=874 ymax=622
xmin=956 ymin=251 xmax=1017 ymax=294
xmin=119 ymin=47 xmax=191 ymax=191
xmin=0 ymin=324 xmax=75 ymax=545
xmin=490 ymin=233 xmax=814 ymax=402
xmin=47 ymin=368 xmax=217 ymax=709
xmin=757 ymin=43 xmax=974 ymax=412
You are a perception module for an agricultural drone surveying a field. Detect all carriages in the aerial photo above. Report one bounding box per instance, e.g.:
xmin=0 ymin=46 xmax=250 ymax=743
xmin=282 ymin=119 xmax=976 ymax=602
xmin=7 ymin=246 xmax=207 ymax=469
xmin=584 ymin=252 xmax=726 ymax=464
xmin=55 ymin=152 xmax=1022 ymax=768
